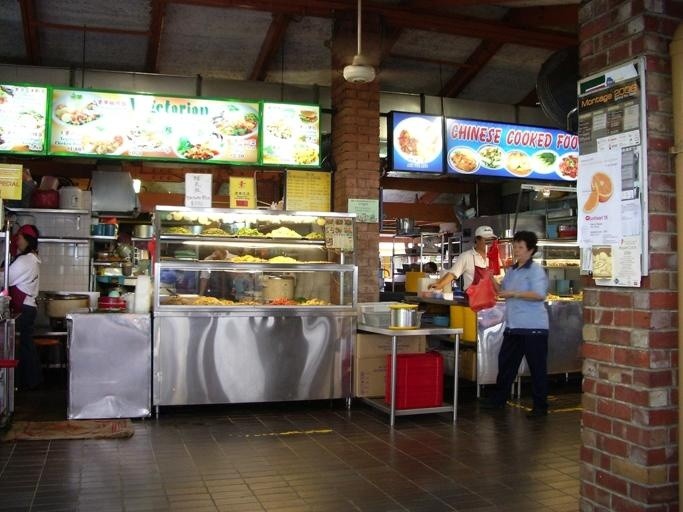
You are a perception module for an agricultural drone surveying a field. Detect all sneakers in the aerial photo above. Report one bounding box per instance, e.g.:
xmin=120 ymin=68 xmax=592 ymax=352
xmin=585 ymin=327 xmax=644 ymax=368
xmin=526 ymin=410 xmax=547 ymax=420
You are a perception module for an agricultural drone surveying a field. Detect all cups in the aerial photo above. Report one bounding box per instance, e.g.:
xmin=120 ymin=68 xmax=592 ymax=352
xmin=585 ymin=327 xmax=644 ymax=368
xmin=96 ymin=223 xmax=114 ymax=235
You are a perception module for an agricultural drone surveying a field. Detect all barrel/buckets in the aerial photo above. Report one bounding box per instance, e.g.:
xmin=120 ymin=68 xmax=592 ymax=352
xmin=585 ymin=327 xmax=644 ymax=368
xmin=395 ymin=215 xmax=415 ymax=235
xmin=135 ymin=274 xmax=151 ymax=313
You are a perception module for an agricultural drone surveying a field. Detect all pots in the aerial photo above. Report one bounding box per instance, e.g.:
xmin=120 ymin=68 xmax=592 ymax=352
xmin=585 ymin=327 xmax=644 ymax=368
xmin=389 ymin=300 xmax=419 ymax=329
xmin=413 ymin=224 xmax=439 ymax=232
xmin=263 ymin=275 xmax=295 ymax=299
xmin=45 ymin=293 xmax=90 ymax=319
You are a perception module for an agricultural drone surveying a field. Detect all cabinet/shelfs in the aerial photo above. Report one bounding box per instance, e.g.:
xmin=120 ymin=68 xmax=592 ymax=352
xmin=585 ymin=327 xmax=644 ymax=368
xmin=355 ymin=196 xmax=582 ymax=427
xmin=0 ymin=204 xmax=352 ymax=422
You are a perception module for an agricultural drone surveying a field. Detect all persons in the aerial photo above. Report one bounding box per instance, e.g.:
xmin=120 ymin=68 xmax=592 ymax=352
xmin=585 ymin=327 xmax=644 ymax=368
xmin=478 ymin=230 xmax=548 ymax=418
xmin=0 ymin=223 xmax=47 ymax=392
xmin=198 ymin=247 xmax=242 ymax=302
xmin=427 ymin=226 xmax=505 ymax=291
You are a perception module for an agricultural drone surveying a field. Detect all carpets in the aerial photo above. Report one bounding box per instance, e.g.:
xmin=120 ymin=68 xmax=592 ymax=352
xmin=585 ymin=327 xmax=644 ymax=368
xmin=2 ymin=416 xmax=133 ymax=445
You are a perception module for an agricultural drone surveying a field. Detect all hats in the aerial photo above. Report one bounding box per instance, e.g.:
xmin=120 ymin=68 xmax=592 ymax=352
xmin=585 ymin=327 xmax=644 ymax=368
xmin=16 ymin=224 xmax=39 ymax=238
xmin=474 ymin=225 xmax=497 ymax=239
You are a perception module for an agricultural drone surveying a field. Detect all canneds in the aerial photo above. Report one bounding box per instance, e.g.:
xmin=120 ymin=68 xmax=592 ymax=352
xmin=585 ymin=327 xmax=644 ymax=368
xmin=97 ymin=223 xmax=115 ymax=236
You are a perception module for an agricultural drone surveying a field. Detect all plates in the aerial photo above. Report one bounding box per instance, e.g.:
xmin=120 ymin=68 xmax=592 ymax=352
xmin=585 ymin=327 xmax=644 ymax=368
xmin=446 ymin=143 xmax=579 ymax=181
xmin=160 ymin=232 xmax=302 ymax=240
xmin=197 ymin=259 xmax=232 ymax=263
xmin=51 ymin=90 xmax=259 ymax=161
xmin=392 ymin=117 xmax=442 ymax=165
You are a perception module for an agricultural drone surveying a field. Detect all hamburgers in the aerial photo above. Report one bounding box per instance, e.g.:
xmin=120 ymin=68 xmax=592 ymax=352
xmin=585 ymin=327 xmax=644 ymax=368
xmin=298 ymin=110 xmax=319 ymax=123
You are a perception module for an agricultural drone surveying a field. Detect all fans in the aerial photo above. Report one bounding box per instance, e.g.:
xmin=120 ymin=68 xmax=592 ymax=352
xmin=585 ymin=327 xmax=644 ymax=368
xmin=274 ymin=0 xmax=453 ymax=89
